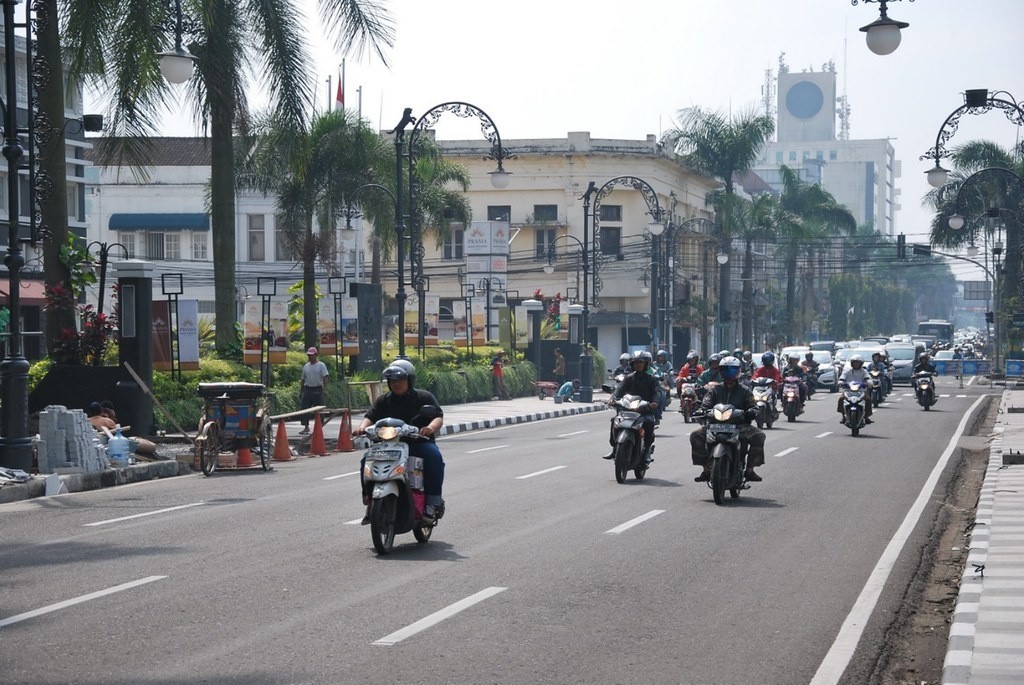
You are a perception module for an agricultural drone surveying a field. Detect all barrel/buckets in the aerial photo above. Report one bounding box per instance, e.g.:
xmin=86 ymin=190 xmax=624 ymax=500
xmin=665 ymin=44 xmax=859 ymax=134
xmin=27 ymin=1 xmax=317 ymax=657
xmin=554 ymin=395 xmax=563 ymax=404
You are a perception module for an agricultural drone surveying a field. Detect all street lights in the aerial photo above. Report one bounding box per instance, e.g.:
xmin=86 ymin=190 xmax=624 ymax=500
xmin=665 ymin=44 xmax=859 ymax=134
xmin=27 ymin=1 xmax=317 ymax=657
xmin=341 ymin=101 xmax=729 ymax=359
xmin=0 ymin=2 xmax=201 ymax=472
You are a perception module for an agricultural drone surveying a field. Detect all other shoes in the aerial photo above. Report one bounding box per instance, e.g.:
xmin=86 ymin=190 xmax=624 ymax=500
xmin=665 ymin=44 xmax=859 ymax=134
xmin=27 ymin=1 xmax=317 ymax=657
xmin=744 ymin=470 xmax=762 ymax=481
xmin=695 ymin=472 xmax=710 ymax=482
xmin=643 ymin=454 xmax=651 ymax=462
xmin=603 ymin=452 xmax=616 ymax=459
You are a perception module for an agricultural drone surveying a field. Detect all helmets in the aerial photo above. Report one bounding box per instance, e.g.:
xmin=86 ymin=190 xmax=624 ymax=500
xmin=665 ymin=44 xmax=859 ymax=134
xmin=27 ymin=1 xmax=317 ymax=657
xmin=806 ymin=352 xmax=813 ymax=360
xmin=687 ymin=352 xmax=699 ymax=362
xmin=762 ymin=352 xmax=775 ymax=367
xmin=709 ymin=350 xmax=730 ymax=365
xmin=307 ymin=347 xmax=317 ymax=356
xmin=656 ymin=350 xmax=668 ymax=361
xmin=719 ymin=356 xmax=742 ymax=379
xmin=380 ymin=360 xmax=416 ymax=387
xmin=851 ymin=354 xmax=865 ymax=369
xmin=919 ymin=352 xmax=929 ymax=362
xmin=619 ymin=350 xmax=653 ymax=368
xmin=872 ymin=350 xmax=887 ymax=361
xmin=788 ymin=352 xmax=801 ymax=364
xmin=733 ymin=348 xmax=753 ymax=363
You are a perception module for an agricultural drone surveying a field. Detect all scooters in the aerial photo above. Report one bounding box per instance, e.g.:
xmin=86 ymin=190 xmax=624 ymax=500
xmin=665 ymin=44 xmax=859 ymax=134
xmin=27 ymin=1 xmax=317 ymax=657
xmin=694 ymin=381 xmax=724 ymax=391
xmin=749 ymin=376 xmax=779 ymax=428
xmin=676 ymin=371 xmax=704 ymax=423
xmin=862 ymin=364 xmax=938 ymax=410
xmin=782 ymin=376 xmax=805 ymax=422
xmin=352 ymin=404 xmax=445 ymax=554
xmin=801 ymin=364 xmax=819 ymax=400
xmin=651 ymin=368 xmax=674 ymax=411
xmin=607 ymin=368 xmax=629 ymax=383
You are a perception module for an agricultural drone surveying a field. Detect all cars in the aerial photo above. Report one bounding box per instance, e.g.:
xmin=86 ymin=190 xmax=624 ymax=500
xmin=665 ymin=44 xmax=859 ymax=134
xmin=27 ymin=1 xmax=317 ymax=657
xmin=749 ymin=340 xmax=850 ymax=392
xmin=843 ymin=319 xmax=977 ymax=385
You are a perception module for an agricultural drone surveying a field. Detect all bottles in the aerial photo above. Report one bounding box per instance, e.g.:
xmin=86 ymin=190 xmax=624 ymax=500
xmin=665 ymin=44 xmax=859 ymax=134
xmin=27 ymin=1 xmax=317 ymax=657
xmin=108 ymin=429 xmax=130 ymax=466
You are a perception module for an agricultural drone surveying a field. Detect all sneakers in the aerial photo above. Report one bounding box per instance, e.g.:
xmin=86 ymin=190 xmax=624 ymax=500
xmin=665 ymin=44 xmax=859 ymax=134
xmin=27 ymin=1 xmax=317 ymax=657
xmin=421 ymin=504 xmax=439 ymax=524
xmin=361 ymin=506 xmax=371 ymax=525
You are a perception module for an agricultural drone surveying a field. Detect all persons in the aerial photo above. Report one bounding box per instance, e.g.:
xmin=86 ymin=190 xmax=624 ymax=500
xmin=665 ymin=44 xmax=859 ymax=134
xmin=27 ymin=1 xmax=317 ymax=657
xmin=490 ymin=349 xmax=513 ymax=401
xmin=86 ymin=400 xmax=118 ymax=433
xmin=553 ymin=330 xmax=982 ymax=421
xmin=299 ymin=347 xmax=329 ymax=434
xmin=602 ymin=350 xmax=664 ymax=461
xmin=352 ymin=360 xmax=444 ymax=527
xmin=690 ymin=356 xmax=766 ymax=482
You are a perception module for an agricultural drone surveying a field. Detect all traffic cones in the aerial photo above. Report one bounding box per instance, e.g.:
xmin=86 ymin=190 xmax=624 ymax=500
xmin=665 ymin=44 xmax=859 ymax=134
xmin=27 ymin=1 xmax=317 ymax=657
xmin=333 ymin=412 xmax=356 ymax=452
xmin=305 ymin=412 xmax=331 ymax=456
xmin=271 ymin=418 xmax=297 ymax=460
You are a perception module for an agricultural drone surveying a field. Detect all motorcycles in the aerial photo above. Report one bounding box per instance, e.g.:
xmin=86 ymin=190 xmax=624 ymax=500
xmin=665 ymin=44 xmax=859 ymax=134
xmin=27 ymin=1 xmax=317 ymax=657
xmin=835 ymin=377 xmax=873 ymax=436
xmin=691 ymin=399 xmax=751 ymax=503
xmin=602 ymin=384 xmax=658 ymax=483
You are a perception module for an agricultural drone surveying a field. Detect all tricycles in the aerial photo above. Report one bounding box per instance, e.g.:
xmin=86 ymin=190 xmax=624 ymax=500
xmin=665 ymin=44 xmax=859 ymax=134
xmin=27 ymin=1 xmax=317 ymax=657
xmin=195 ymin=393 xmax=273 ymax=477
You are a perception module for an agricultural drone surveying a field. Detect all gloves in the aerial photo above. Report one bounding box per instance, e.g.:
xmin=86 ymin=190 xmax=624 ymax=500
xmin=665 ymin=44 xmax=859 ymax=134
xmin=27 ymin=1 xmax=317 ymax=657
xmin=747 ymin=407 xmax=761 ymax=419
xmin=696 ymin=407 xmax=704 ymax=416
